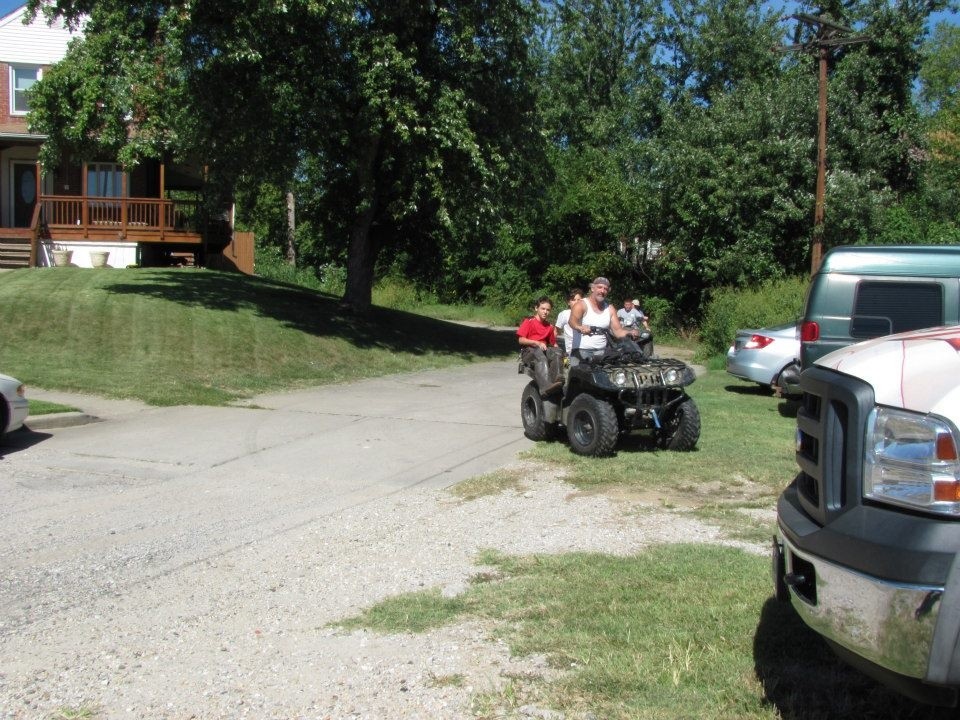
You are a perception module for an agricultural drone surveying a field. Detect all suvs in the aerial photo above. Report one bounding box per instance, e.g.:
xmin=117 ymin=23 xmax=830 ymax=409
xmin=619 ymin=317 xmax=653 ymax=356
xmin=517 ymin=326 xmax=702 ymax=459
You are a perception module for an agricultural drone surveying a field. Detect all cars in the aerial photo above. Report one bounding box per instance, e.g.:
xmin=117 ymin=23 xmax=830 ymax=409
xmin=725 ymin=320 xmax=804 ymax=400
xmin=0 ymin=373 xmax=29 ymax=438
xmin=772 ymin=325 xmax=960 ymax=712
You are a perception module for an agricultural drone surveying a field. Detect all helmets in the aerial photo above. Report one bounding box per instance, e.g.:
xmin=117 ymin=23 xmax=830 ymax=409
xmin=631 ymin=299 xmax=640 ymax=306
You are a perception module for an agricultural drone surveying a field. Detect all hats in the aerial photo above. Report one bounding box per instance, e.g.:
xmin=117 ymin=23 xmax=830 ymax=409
xmin=593 ymin=277 xmax=610 ymax=286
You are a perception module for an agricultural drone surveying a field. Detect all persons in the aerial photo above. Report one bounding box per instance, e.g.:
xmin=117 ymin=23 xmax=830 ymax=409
xmin=633 ymin=300 xmax=651 ymax=331
xmin=554 ymin=289 xmax=583 ymax=356
xmin=516 ymin=296 xmax=565 ymax=398
xmin=617 ymin=299 xmax=648 ymax=329
xmin=568 ymin=277 xmax=639 ymax=366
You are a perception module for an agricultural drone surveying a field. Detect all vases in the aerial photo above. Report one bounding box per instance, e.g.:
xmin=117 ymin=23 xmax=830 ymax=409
xmin=52 ymin=249 xmax=73 ymax=266
xmin=91 ymin=251 xmax=110 ymax=268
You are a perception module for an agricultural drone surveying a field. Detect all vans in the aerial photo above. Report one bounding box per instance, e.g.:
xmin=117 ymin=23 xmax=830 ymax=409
xmin=782 ymin=244 xmax=960 ymax=399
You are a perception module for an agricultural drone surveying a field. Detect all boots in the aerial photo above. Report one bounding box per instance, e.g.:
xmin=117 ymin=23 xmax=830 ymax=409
xmin=549 ymin=355 xmax=565 ymax=386
xmin=534 ymin=360 xmax=563 ymax=400
